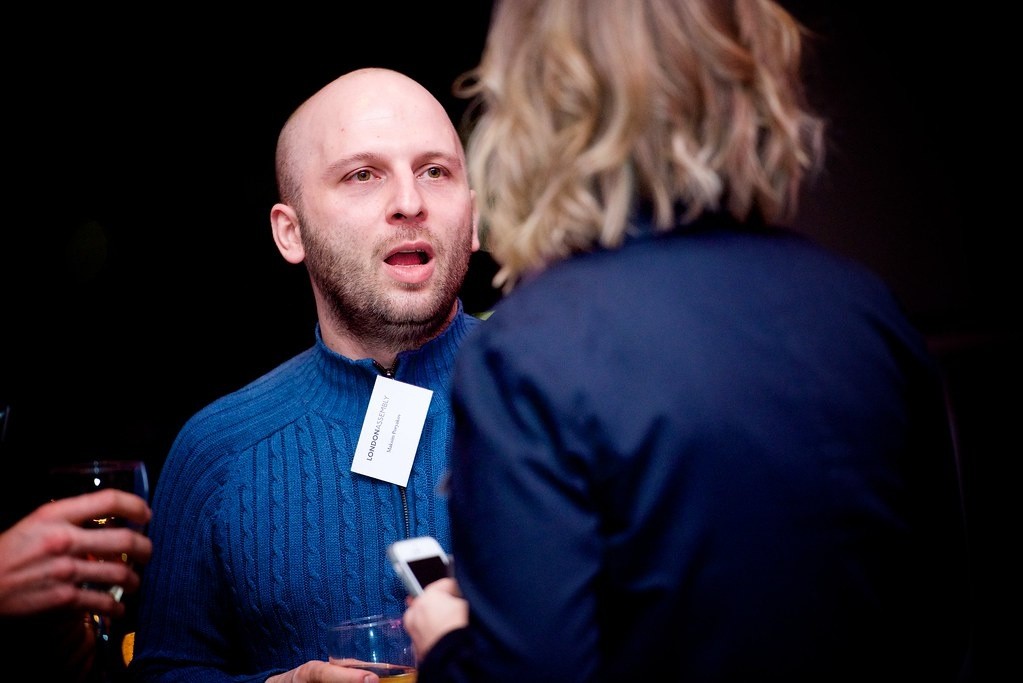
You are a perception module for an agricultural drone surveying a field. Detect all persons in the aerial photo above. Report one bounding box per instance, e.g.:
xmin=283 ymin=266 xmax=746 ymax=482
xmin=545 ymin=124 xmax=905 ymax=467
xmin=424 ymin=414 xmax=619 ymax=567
xmin=403 ymin=1 xmax=967 ymax=683
xmin=137 ymin=67 xmax=488 ymax=683
xmin=0 ymin=489 xmax=152 ymax=611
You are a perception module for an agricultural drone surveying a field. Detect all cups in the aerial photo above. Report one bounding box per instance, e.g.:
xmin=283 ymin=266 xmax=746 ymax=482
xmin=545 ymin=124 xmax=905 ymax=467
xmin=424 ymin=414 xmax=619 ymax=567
xmin=326 ymin=612 xmax=420 ymax=683
xmin=46 ymin=458 xmax=151 ymax=644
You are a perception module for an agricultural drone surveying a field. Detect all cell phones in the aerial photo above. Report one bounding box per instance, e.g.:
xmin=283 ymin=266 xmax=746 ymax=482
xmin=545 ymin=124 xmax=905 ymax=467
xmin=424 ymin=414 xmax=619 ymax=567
xmin=387 ymin=536 xmax=450 ymax=596
